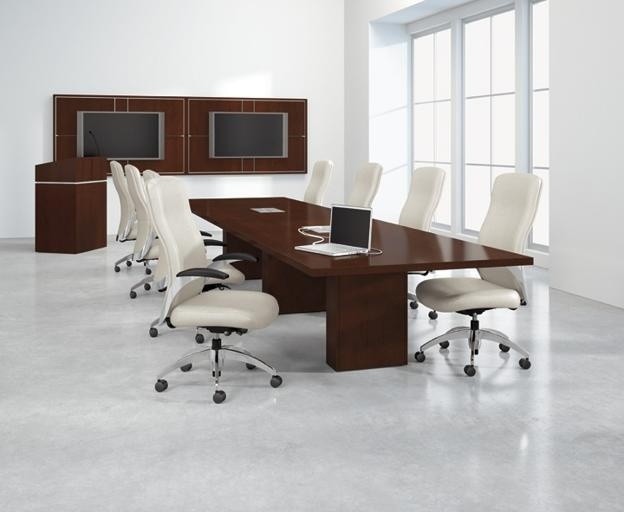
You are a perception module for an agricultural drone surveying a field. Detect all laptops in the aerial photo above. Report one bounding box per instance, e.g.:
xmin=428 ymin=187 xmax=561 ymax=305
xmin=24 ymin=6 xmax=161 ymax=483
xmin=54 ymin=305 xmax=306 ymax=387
xmin=294 ymin=205 xmax=373 ymax=257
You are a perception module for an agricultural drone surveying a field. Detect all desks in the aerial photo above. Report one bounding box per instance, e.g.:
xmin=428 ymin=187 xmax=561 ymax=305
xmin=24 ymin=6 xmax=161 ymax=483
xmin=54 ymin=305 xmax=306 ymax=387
xmin=191 ymin=195 xmax=532 ymax=374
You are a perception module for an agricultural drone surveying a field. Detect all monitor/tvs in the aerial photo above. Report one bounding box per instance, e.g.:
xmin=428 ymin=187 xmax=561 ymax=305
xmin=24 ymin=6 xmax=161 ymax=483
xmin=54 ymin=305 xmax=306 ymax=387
xmin=209 ymin=112 xmax=289 ymax=159
xmin=76 ymin=111 xmax=166 ymax=161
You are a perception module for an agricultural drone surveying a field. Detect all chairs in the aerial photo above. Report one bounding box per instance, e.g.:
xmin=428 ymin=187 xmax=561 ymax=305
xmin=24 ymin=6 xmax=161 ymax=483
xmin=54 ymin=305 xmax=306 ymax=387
xmin=399 ymin=164 xmax=447 ymax=321
xmin=412 ymin=170 xmax=545 ymax=378
xmin=303 ymin=159 xmax=336 ymax=205
xmin=107 ymin=160 xmax=231 ymax=300
xmin=346 ymin=161 xmax=385 ymax=208
xmin=144 ymin=175 xmax=283 ymax=406
